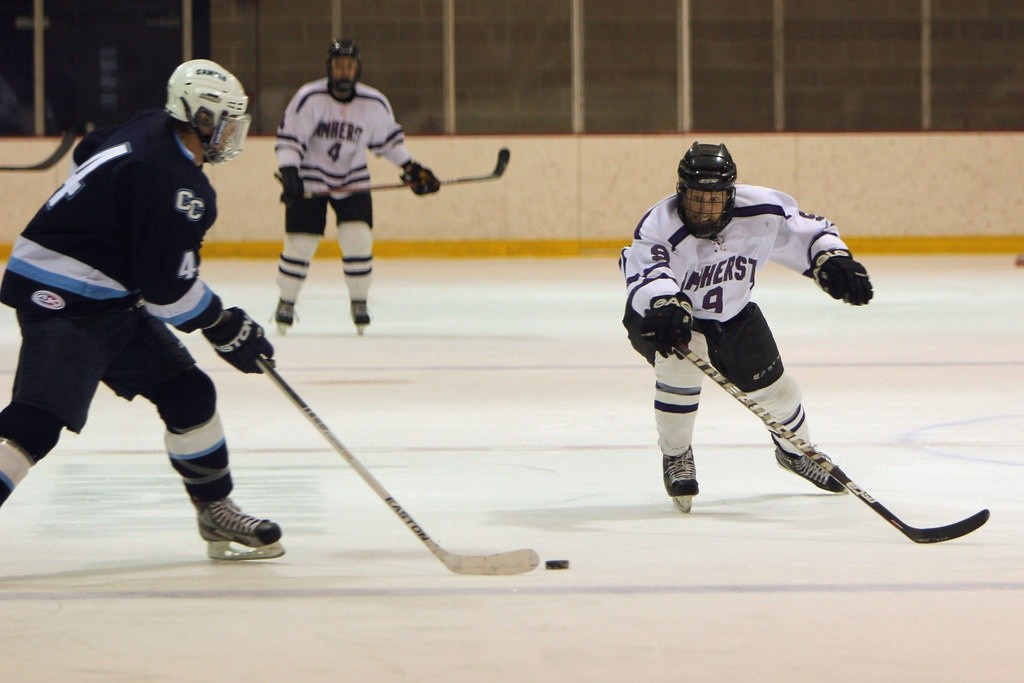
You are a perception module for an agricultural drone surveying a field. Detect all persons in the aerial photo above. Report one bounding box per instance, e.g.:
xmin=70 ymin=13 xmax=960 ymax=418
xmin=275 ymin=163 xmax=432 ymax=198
xmin=274 ymin=38 xmax=440 ymax=336
xmin=0 ymin=59 xmax=287 ymax=561
xmin=618 ymin=141 xmax=874 ymax=514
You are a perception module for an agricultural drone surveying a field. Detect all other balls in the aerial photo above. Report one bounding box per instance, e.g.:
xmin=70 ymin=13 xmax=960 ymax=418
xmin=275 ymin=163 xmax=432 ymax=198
xmin=545 ymin=558 xmax=569 ymax=570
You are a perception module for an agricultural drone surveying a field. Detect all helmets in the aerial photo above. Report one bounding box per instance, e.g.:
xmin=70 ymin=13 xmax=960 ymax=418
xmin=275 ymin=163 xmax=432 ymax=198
xmin=325 ymin=38 xmax=362 ymax=94
xmin=675 ymin=141 xmax=737 ymax=239
xmin=163 ymin=58 xmax=249 ymax=127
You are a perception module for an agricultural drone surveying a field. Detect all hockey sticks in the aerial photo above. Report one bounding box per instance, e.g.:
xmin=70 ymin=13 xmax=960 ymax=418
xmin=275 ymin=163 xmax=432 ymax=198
xmin=251 ymin=354 xmax=540 ymax=578
xmin=667 ymin=338 xmax=994 ymax=544
xmin=306 ymin=143 xmax=511 ymax=201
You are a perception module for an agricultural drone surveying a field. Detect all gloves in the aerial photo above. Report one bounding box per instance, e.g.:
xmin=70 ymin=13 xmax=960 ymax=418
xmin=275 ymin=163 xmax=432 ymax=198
xmin=812 ymin=248 xmax=873 ymax=306
xmin=641 ymin=292 xmax=694 ymax=359
xmin=281 ymin=167 xmax=304 ymax=218
xmin=399 ymin=163 xmax=441 ymax=195
xmin=202 ymin=307 xmax=276 ymax=374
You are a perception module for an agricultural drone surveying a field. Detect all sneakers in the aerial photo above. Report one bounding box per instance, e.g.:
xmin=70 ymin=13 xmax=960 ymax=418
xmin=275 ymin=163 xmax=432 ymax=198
xmin=775 ymin=446 xmax=850 ymax=494
xmin=351 ymin=300 xmax=370 ymax=336
xmin=275 ymin=298 xmax=295 ymax=337
xmin=663 ymin=444 xmax=699 ymax=514
xmin=197 ymin=498 xmax=286 ymax=561
